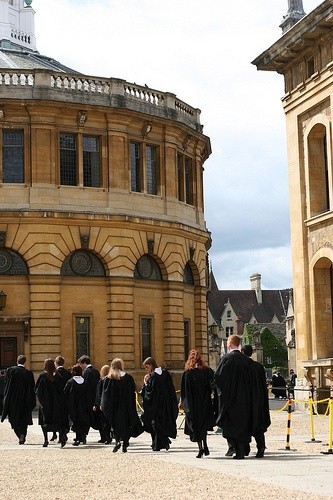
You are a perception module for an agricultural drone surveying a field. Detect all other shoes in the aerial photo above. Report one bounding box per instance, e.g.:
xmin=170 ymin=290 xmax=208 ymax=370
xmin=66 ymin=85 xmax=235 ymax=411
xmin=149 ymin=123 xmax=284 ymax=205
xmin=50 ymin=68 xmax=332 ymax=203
xmin=43 ymin=441 xmax=48 ymax=447
xmin=58 ymin=434 xmax=68 ymax=447
xmin=150 ymin=437 xmax=171 ymax=451
xmin=204 ymin=447 xmax=209 ymax=456
xmin=255 ymin=447 xmax=264 ymax=458
xmin=123 ymin=440 xmax=130 ymax=453
xmin=19 ymin=434 xmax=25 ymax=444
xmin=196 ymin=449 xmax=204 ymax=458
xmin=50 ymin=435 xmax=58 ymax=441
xmin=113 ymin=443 xmax=121 ymax=452
xmin=98 ymin=438 xmax=105 ymax=443
xmin=73 ymin=436 xmax=86 ymax=446
xmin=105 ymin=437 xmax=112 ymax=444
xmin=225 ymin=445 xmax=250 ymax=459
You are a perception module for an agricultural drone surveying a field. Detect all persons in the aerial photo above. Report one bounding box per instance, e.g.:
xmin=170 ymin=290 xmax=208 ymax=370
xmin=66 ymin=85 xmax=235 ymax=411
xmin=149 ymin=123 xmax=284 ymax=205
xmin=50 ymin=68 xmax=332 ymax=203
xmin=33 ymin=357 xmax=70 ymax=448
xmin=98 ymin=358 xmax=143 ymax=453
xmin=70 ymin=355 xmax=98 ymax=441
xmin=140 ymin=357 xmax=179 ymax=451
xmin=239 ymin=344 xmax=271 ymax=458
xmin=49 ymin=356 xmax=72 ymax=444
xmin=180 ymin=349 xmax=217 ymax=458
xmin=92 ymin=364 xmax=112 ymax=444
xmin=271 ymin=369 xmax=296 ymax=400
xmin=63 ymin=365 xmax=92 ymax=446
xmin=0 ymin=354 xmax=36 ymax=444
xmin=213 ymin=334 xmax=258 ymax=460
